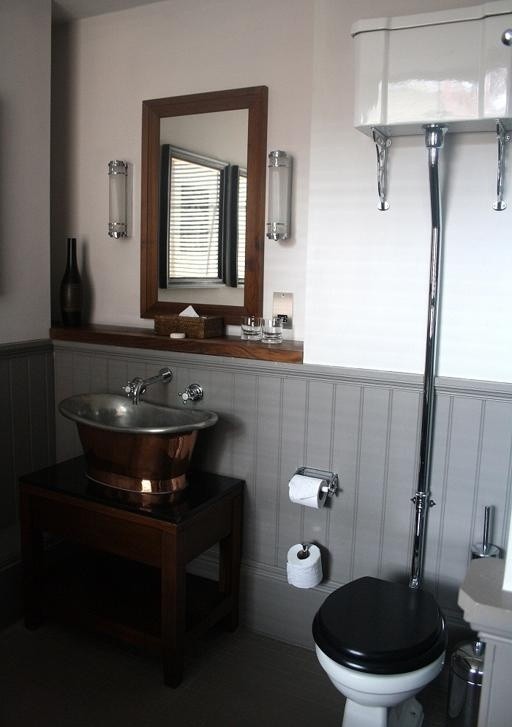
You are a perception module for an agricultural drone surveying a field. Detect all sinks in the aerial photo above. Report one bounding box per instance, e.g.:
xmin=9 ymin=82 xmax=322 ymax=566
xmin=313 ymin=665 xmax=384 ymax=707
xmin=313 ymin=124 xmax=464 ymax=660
xmin=57 ymin=392 xmax=217 ymax=495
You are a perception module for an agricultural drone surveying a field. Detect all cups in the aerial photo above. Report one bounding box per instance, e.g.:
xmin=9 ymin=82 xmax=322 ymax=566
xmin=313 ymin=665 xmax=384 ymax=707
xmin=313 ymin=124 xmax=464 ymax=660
xmin=240 ymin=316 xmax=283 ymax=345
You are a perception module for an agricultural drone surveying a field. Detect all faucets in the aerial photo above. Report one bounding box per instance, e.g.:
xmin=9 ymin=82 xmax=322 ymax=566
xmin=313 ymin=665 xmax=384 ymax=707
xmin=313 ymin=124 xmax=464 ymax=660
xmin=121 ymin=367 xmax=171 ymax=406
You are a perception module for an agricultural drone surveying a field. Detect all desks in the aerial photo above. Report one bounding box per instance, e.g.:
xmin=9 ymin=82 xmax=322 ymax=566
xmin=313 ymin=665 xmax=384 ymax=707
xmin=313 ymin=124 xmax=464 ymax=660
xmin=17 ymin=461 xmax=245 ymax=688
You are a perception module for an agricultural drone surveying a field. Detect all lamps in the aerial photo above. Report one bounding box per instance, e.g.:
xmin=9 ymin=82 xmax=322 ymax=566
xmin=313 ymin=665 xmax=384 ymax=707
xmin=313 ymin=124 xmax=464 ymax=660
xmin=266 ymin=151 xmax=290 ymax=241
xmin=108 ymin=160 xmax=128 ymax=239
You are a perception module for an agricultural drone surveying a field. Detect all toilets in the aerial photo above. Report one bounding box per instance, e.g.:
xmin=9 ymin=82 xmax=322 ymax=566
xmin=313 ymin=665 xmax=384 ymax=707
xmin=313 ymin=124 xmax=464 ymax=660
xmin=311 ymin=572 xmax=449 ymax=727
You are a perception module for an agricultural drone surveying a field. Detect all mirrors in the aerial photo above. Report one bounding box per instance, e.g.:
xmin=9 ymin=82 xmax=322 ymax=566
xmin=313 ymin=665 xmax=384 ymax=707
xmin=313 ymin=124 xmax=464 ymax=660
xmin=140 ymin=86 xmax=269 ymax=319
